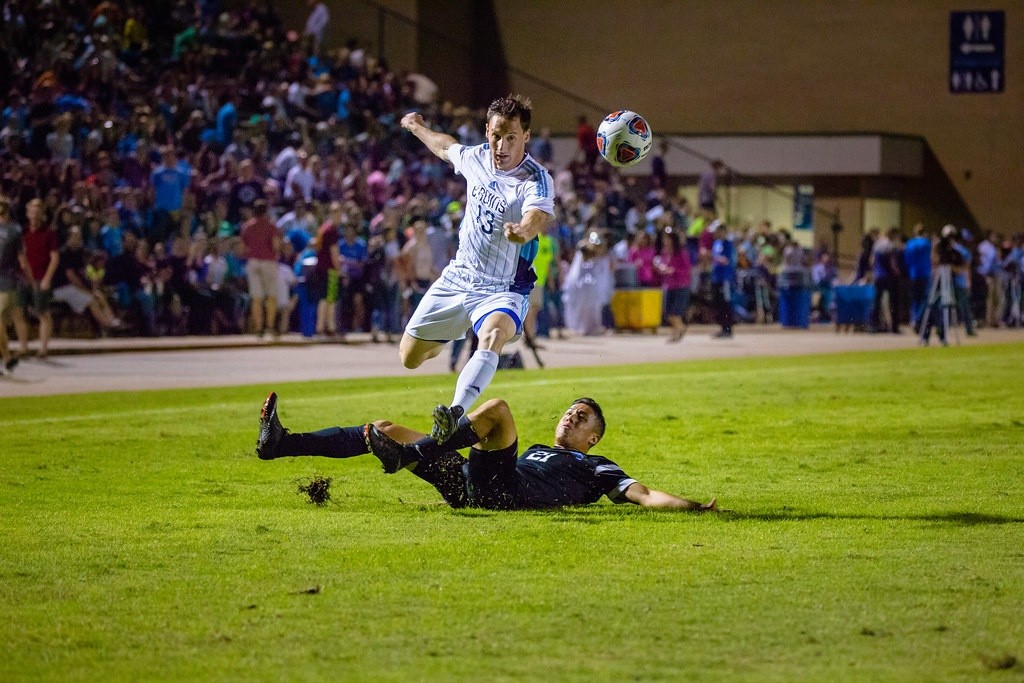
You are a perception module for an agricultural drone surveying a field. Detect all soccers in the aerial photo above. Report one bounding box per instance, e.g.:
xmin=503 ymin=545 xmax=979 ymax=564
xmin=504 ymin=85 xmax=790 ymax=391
xmin=594 ymin=107 xmax=655 ymax=170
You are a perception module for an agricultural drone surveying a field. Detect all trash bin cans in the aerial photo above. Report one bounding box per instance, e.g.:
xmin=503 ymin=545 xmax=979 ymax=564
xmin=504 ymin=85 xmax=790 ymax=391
xmin=608 ymin=281 xmax=664 ymax=335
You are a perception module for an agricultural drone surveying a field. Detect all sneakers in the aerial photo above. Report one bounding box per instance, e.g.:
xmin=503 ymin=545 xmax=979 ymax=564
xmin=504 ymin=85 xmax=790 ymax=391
xmin=256 ymin=391 xmax=290 ymax=460
xmin=363 ymin=423 xmax=412 ymax=474
xmin=430 ymin=404 xmax=464 ymax=445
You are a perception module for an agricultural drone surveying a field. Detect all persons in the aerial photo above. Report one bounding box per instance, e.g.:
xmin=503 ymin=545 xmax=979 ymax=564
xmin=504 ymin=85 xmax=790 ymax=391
xmin=401 ymin=93 xmax=555 ymax=442
xmin=255 ymin=392 xmax=716 ymax=512
xmin=0 ymin=0 xmax=1024 ymax=372
